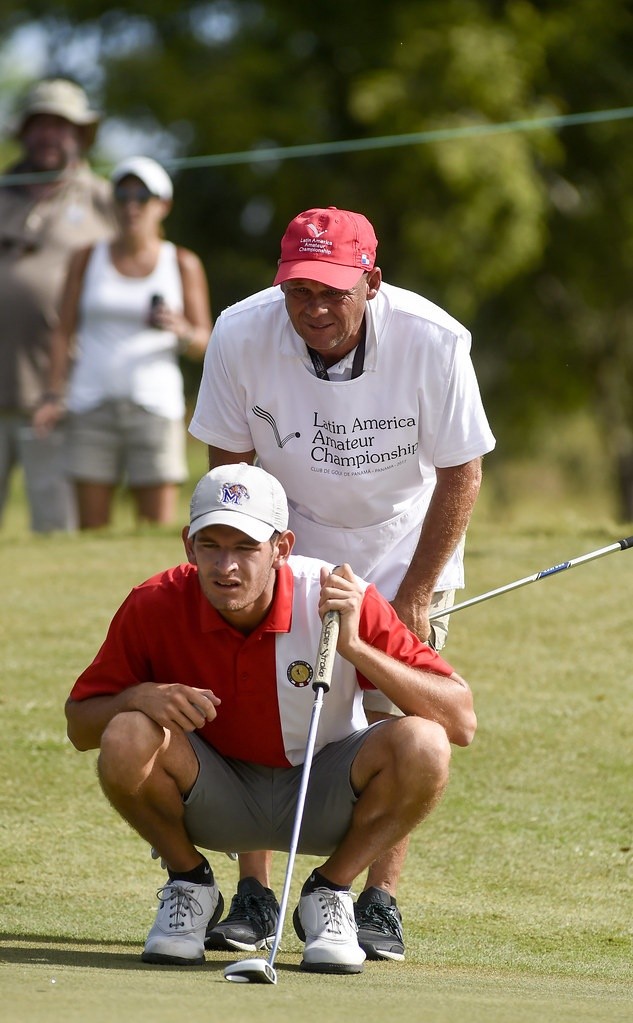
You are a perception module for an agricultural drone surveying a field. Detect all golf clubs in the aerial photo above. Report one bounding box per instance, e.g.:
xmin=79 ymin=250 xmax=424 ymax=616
xmin=428 ymin=535 xmax=632 ymax=621
xmin=221 ymin=567 xmax=344 ymax=986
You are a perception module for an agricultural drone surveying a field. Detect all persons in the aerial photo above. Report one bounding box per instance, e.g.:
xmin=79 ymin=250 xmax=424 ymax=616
xmin=0 ymin=76 xmax=115 ymax=534
xmin=23 ymin=155 xmax=211 ymax=531
xmin=182 ymin=205 xmax=497 ymax=959
xmin=65 ymin=460 xmax=478 ymax=974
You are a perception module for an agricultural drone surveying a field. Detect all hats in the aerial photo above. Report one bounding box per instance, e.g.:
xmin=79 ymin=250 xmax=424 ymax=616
xmin=274 ymin=204 xmax=378 ymax=290
xmin=189 ymin=462 xmax=289 ymax=543
xmin=2 ymin=78 xmax=104 ymax=153
xmin=110 ymin=155 xmax=173 ymax=199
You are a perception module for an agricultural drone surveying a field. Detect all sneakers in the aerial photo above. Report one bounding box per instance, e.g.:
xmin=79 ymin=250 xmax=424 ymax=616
xmin=292 ymin=869 xmax=365 ymax=974
xmin=204 ymin=877 xmax=281 ymax=951
xmin=142 ymin=862 xmax=224 ymax=964
xmin=352 ymin=886 xmax=406 ymax=962
